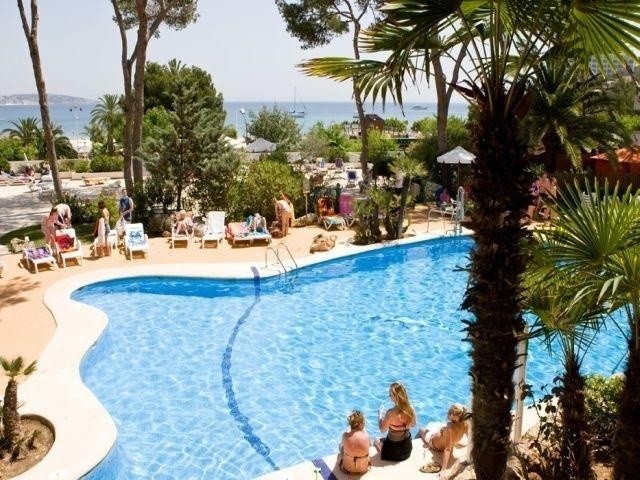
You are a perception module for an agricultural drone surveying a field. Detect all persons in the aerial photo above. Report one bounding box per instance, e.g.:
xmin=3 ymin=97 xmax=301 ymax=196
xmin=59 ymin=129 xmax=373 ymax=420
xmin=527 ymin=172 xmax=557 ymax=220
xmin=338 ymin=410 xmax=371 ymax=476
xmin=247 ymin=194 xmax=294 ymax=239
xmin=24 ymin=162 xmax=50 ymax=183
xmin=417 ymin=403 xmax=470 ymax=479
xmin=374 ymin=382 xmax=416 ymax=461
xmin=42 ymin=189 xmax=193 ymax=259
xmin=395 ymin=176 xmax=450 ymax=211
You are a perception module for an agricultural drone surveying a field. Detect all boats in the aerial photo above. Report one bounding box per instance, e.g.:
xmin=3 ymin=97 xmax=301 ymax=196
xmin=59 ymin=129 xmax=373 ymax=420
xmin=353 ymin=110 xmax=360 ymax=119
xmin=412 ymin=104 xmax=427 ymax=110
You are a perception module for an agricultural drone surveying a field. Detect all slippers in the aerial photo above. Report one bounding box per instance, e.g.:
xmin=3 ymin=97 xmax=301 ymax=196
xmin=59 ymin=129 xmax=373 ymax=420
xmin=428 ymin=461 xmax=441 ymax=471
xmin=421 ymin=466 xmax=439 ymax=473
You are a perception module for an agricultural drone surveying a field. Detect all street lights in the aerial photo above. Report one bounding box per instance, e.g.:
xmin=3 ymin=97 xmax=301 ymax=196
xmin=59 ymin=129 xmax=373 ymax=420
xmin=239 ymin=107 xmax=251 ymax=147
xmin=69 ymin=104 xmax=83 ymax=150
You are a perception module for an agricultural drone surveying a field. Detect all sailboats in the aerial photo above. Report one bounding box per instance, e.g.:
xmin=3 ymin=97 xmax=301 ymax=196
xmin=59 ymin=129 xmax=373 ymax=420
xmin=285 ymin=86 xmax=308 ymax=119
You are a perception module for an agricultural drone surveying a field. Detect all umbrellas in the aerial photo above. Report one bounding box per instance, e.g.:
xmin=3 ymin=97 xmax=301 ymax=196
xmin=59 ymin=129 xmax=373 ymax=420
xmin=437 ymin=146 xmax=477 ymax=186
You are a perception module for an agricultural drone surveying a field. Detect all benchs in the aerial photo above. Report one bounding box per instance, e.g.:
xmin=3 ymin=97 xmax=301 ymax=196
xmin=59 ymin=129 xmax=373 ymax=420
xmin=125 ymin=222 xmax=149 ymax=260
xmin=202 ymin=211 xmax=224 ymax=248
xmin=315 ymin=204 xmax=347 ymax=230
xmin=22 ymin=243 xmax=54 ymax=271
xmin=426 ymin=197 xmax=459 ymax=220
xmin=229 ymin=222 xmax=270 ymax=247
xmin=171 ymin=211 xmax=194 ymax=249
xmin=93 ymin=229 xmax=118 ymax=257
xmin=54 ymin=228 xmax=83 ymax=268
